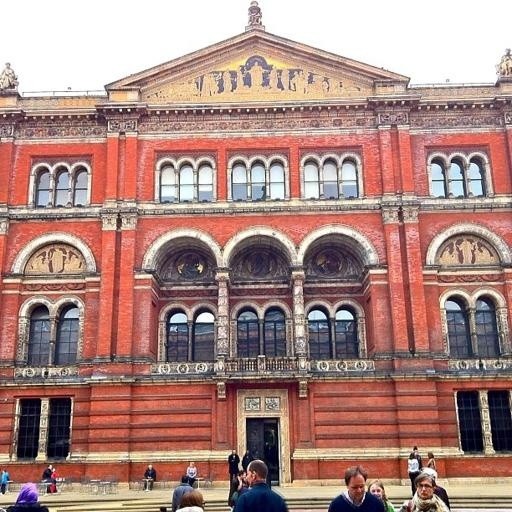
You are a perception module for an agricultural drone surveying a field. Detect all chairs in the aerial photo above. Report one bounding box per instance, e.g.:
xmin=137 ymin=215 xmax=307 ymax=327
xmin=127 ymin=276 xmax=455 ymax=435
xmin=3 ymin=476 xmax=215 ymax=496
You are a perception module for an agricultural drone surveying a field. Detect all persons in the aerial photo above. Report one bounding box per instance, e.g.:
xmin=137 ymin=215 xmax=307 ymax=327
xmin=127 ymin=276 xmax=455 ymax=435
xmin=399 ymin=473 xmax=449 ymax=512
xmin=407 ymin=453 xmax=420 ymax=496
xmin=420 ymin=467 xmax=450 ymax=510
xmin=172 ymin=474 xmax=193 ymax=512
xmin=0 ymin=470 xmax=9 ymax=494
xmin=6 ymin=482 xmax=49 ymax=512
xmin=367 ymin=479 xmax=396 ymax=512
xmin=427 ymin=452 xmax=436 ymax=470
xmin=42 ymin=464 xmax=56 ymax=493
xmin=175 ymin=491 xmax=204 ymax=512
xmin=49 ymin=467 xmax=57 ymax=493
xmin=328 ymin=465 xmax=384 ymax=512
xmin=228 ymin=449 xmax=291 ymax=512
xmin=142 ymin=464 xmax=156 ymax=490
xmin=413 ymin=446 xmax=422 ymax=470
xmin=187 ymin=461 xmax=198 ymax=487
